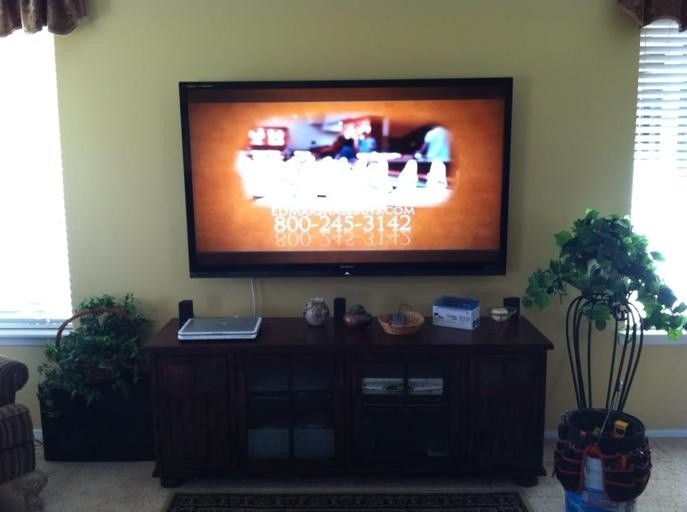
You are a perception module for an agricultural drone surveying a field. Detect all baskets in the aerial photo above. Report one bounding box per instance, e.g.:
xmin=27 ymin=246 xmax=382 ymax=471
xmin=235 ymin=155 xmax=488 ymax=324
xmin=376 ymin=303 xmax=426 ymax=336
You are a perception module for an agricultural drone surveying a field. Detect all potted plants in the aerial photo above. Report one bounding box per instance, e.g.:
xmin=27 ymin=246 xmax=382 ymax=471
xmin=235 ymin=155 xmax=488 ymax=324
xmin=36 ymin=291 xmax=156 ymax=418
xmin=522 ymin=208 xmax=687 ymax=340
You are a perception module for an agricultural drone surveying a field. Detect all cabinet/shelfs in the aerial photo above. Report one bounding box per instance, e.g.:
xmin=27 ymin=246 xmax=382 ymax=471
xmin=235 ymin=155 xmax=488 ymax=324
xmin=144 ymin=317 xmax=553 ymax=487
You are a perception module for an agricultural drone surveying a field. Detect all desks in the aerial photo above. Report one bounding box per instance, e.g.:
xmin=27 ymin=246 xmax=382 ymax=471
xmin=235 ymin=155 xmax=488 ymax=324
xmin=39 ymin=373 xmax=154 ymax=461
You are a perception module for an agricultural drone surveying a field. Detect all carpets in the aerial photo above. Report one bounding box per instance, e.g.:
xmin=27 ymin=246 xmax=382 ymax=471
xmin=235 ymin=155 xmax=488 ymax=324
xmin=167 ymin=492 xmax=529 ymax=512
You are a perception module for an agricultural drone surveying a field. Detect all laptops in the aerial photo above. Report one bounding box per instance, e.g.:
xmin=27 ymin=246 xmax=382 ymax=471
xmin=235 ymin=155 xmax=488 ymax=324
xmin=177 ymin=317 xmax=262 ymax=341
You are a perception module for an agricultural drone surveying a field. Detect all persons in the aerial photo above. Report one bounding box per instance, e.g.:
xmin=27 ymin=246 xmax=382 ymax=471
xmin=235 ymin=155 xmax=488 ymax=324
xmin=283 ymin=118 xmax=450 ymax=162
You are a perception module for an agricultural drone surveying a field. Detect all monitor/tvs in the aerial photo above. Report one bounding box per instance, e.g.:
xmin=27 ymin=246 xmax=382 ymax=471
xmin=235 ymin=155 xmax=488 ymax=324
xmin=178 ymin=75 xmax=514 ymax=280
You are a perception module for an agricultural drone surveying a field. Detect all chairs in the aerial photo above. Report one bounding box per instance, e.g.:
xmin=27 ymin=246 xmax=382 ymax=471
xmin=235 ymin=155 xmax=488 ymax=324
xmin=0 ymin=354 xmax=48 ymax=512
